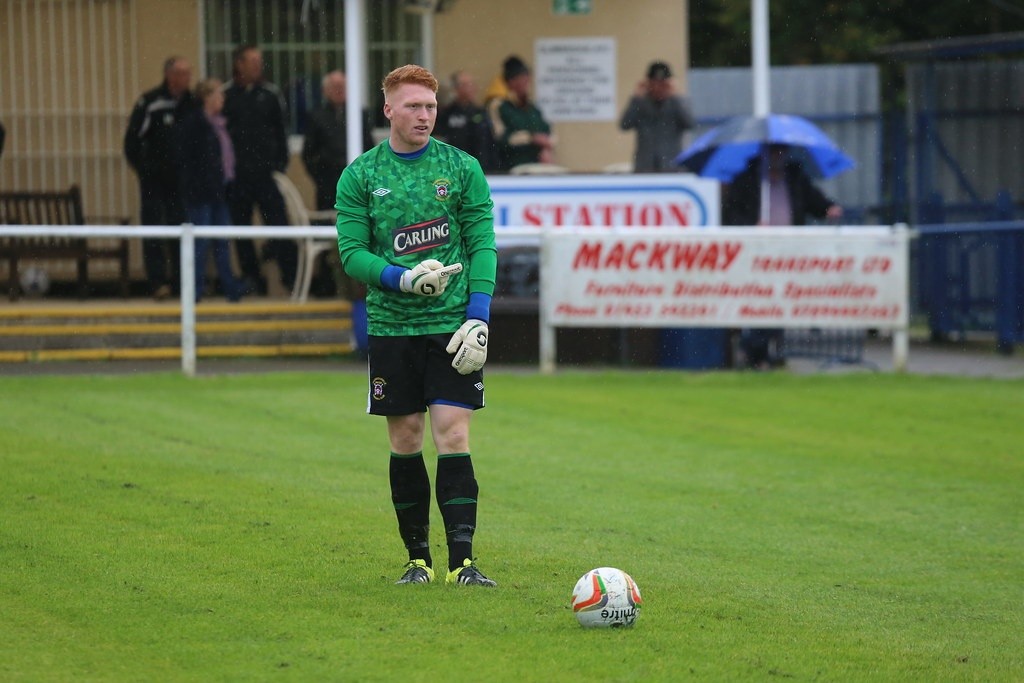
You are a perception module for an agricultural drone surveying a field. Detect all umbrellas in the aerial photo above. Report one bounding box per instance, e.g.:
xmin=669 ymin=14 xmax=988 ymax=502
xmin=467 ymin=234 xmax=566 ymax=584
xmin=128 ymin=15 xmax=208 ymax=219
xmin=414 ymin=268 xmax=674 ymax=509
xmin=675 ymin=112 xmax=855 ymax=183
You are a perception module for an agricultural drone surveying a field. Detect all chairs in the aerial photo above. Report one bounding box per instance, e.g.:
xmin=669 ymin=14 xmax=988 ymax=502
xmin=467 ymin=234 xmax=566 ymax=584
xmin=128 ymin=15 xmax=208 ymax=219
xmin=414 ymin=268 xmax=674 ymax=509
xmin=273 ymin=173 xmax=344 ymax=302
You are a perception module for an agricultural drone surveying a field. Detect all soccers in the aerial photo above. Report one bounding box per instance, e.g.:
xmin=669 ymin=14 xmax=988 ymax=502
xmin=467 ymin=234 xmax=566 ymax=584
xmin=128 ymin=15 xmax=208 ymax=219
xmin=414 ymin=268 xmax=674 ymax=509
xmin=572 ymin=566 xmax=642 ymax=630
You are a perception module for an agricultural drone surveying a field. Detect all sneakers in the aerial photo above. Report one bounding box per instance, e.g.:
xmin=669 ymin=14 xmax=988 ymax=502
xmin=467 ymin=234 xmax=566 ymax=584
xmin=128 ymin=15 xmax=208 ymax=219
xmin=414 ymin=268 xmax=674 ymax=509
xmin=445 ymin=558 xmax=497 ymax=587
xmin=395 ymin=559 xmax=435 ymax=585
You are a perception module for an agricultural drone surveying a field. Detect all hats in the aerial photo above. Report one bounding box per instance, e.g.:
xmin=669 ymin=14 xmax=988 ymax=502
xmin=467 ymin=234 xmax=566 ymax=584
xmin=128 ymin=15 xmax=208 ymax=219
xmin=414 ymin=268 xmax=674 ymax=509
xmin=502 ymin=55 xmax=529 ymax=82
xmin=647 ymin=61 xmax=672 ymax=80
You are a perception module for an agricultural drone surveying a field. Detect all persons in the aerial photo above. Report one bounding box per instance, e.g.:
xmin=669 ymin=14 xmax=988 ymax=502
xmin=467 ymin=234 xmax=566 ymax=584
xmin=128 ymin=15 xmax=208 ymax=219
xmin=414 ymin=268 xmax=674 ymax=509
xmin=435 ymin=69 xmax=499 ymax=175
xmin=731 ymin=144 xmax=844 ymax=367
xmin=123 ymin=44 xmax=298 ymax=299
xmin=618 ymin=61 xmax=694 ymax=172
xmin=334 ymin=65 xmax=497 ymax=586
xmin=301 ymin=71 xmax=388 ymax=297
xmin=484 ymin=55 xmax=552 ymax=170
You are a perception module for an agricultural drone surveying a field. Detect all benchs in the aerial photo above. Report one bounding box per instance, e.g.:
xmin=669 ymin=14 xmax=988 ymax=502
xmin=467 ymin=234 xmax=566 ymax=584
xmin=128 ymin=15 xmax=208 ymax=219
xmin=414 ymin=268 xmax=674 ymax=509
xmin=0 ymin=187 xmax=130 ymax=298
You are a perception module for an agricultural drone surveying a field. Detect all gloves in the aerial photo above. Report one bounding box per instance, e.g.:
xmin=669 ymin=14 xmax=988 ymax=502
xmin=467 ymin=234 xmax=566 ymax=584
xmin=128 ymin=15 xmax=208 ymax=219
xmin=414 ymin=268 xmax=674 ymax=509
xmin=445 ymin=318 xmax=488 ymax=375
xmin=399 ymin=259 xmax=463 ymax=297
